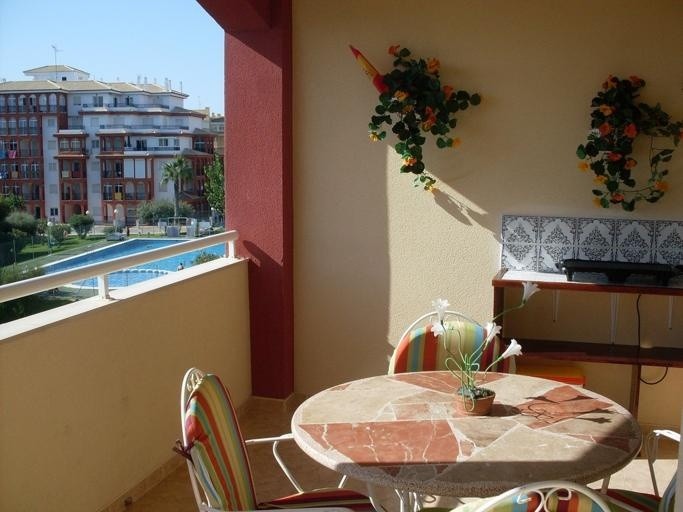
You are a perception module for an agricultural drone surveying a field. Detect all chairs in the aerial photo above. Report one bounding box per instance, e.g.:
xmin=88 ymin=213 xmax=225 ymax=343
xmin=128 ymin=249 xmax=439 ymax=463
xmin=484 ymin=428 xmax=680 ymax=512
xmin=180 ymin=368 xmax=385 ymax=512
xmin=386 ymin=311 xmax=516 ymax=510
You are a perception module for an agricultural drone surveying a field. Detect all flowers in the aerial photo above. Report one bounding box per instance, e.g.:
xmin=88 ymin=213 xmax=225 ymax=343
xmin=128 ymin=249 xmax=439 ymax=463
xmin=421 ymin=280 xmax=542 ymax=412
xmin=577 ymin=74 xmax=682 ymax=212
xmin=361 ymin=43 xmax=482 ymax=192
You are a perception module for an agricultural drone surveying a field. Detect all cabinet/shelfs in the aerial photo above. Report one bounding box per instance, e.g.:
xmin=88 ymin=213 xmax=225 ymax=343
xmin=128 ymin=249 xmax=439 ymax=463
xmin=492 ymin=266 xmax=682 ymax=421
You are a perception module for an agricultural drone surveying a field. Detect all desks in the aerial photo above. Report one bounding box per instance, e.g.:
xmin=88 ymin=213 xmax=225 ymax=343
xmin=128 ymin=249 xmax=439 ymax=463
xmin=292 ymin=369 xmax=646 ymax=511
